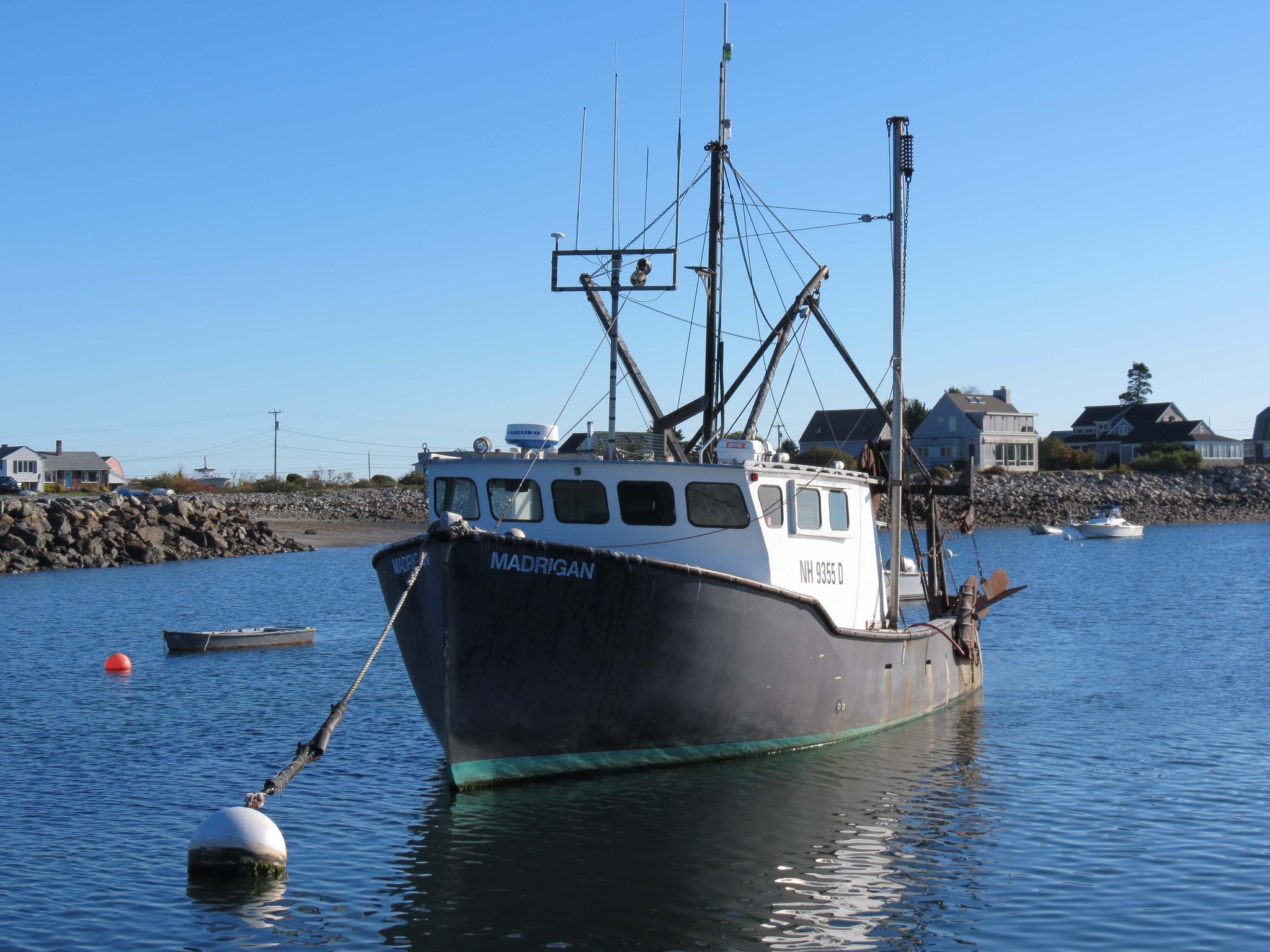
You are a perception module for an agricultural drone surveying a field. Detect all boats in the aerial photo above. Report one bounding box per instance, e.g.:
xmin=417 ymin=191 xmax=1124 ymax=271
xmin=193 ymin=454 xmax=231 ymax=489
xmin=369 ymin=0 xmax=1027 ymax=794
xmin=1066 ymin=498 xmax=1145 ymax=538
xmin=1026 ymin=523 xmax=1064 ymax=535
xmin=161 ymin=624 xmax=316 ymax=652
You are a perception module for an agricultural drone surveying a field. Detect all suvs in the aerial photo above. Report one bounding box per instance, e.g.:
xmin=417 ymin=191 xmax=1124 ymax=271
xmin=0 ymin=476 xmax=23 ymax=492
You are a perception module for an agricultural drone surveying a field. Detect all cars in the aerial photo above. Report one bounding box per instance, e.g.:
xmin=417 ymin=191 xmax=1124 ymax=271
xmin=110 ymin=486 xmax=124 ymax=493
